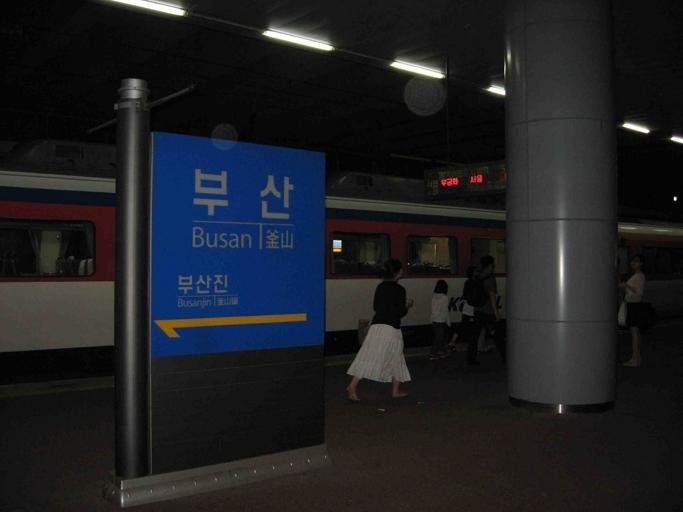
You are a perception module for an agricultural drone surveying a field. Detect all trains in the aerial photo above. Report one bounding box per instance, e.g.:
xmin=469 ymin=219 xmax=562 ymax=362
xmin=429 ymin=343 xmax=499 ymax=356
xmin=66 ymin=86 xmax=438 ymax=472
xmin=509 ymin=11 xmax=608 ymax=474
xmin=0 ymin=136 xmax=683 ymax=354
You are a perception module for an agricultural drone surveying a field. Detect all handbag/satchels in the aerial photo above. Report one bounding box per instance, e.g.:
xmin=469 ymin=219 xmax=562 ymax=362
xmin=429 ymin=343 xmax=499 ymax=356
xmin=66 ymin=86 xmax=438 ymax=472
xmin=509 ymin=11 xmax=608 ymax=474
xmin=616 ymin=297 xmax=626 ymax=326
xmin=357 ymin=318 xmax=371 ymax=347
xmin=446 ymin=303 xmax=462 ymax=323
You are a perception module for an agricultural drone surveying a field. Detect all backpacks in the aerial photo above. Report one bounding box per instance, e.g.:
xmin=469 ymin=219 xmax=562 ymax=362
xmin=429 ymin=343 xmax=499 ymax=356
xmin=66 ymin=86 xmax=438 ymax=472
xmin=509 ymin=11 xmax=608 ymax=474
xmin=463 ymin=270 xmax=493 ymax=307
xmin=625 ymin=299 xmax=656 ymax=329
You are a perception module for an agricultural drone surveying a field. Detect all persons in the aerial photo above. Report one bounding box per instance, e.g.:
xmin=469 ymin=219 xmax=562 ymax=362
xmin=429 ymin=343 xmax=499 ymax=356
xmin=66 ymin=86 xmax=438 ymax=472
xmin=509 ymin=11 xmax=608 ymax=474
xmin=448 ymin=254 xmax=505 ymax=366
xmin=344 ymin=258 xmax=414 ymax=402
xmin=618 ymin=254 xmax=646 ymax=368
xmin=428 ymin=279 xmax=451 ymax=360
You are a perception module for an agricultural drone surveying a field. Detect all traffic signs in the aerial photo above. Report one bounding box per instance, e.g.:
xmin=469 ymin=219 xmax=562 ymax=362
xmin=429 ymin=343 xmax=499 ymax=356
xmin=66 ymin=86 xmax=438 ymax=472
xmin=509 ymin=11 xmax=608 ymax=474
xmin=149 ymin=130 xmax=326 ymax=356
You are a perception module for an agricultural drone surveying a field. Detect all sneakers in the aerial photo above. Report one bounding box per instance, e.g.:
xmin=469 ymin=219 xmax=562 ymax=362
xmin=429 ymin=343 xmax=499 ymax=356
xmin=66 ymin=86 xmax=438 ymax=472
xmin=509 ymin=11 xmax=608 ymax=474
xmin=391 ymin=390 xmax=408 ymax=399
xmin=344 ymin=384 xmax=359 ymax=403
xmin=622 ymin=357 xmax=641 ymax=368
xmin=427 ymin=343 xmax=489 ymax=360
